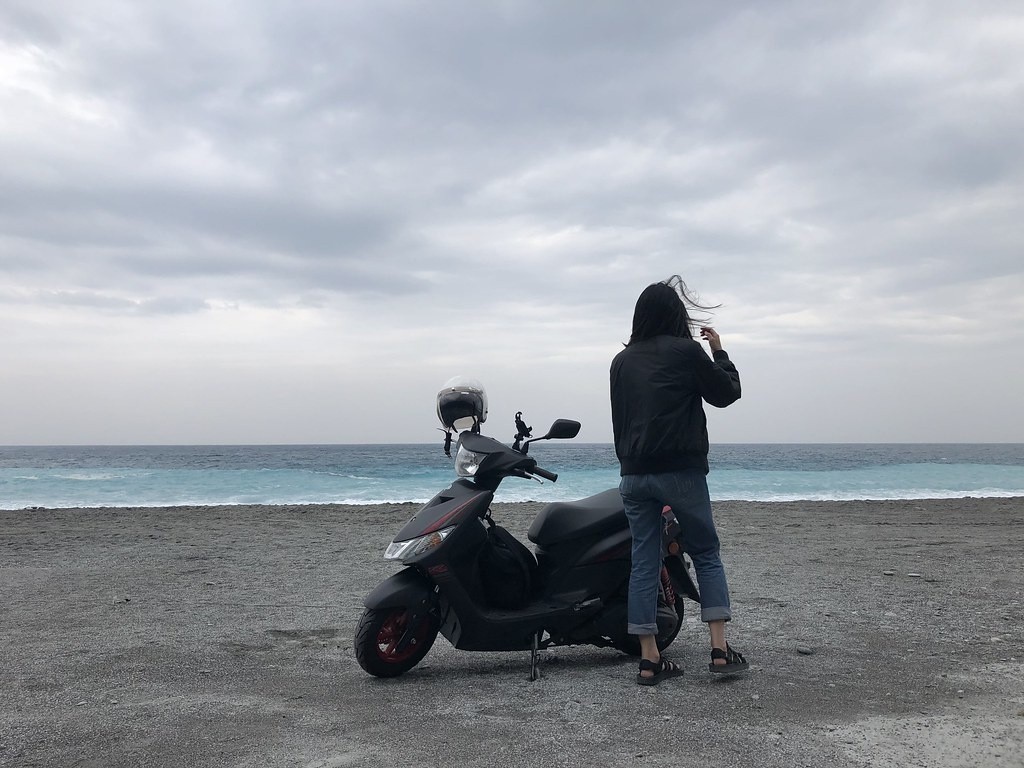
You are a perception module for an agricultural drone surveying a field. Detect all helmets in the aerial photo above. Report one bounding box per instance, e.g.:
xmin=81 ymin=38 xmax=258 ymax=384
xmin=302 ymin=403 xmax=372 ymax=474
xmin=435 ymin=374 xmax=489 ymax=428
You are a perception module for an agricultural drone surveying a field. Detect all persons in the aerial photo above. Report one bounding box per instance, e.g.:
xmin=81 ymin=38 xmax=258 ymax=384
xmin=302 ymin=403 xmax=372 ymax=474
xmin=609 ymin=275 xmax=751 ymax=685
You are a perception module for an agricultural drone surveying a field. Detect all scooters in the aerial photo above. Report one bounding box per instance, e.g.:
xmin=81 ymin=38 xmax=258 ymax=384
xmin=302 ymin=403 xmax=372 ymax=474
xmin=353 ymin=411 xmax=704 ymax=680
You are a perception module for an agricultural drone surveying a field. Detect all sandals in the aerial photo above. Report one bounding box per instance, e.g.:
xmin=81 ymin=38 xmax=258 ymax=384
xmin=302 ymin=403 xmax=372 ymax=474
xmin=709 ymin=640 xmax=749 ymax=674
xmin=636 ymin=652 xmax=685 ymax=686
